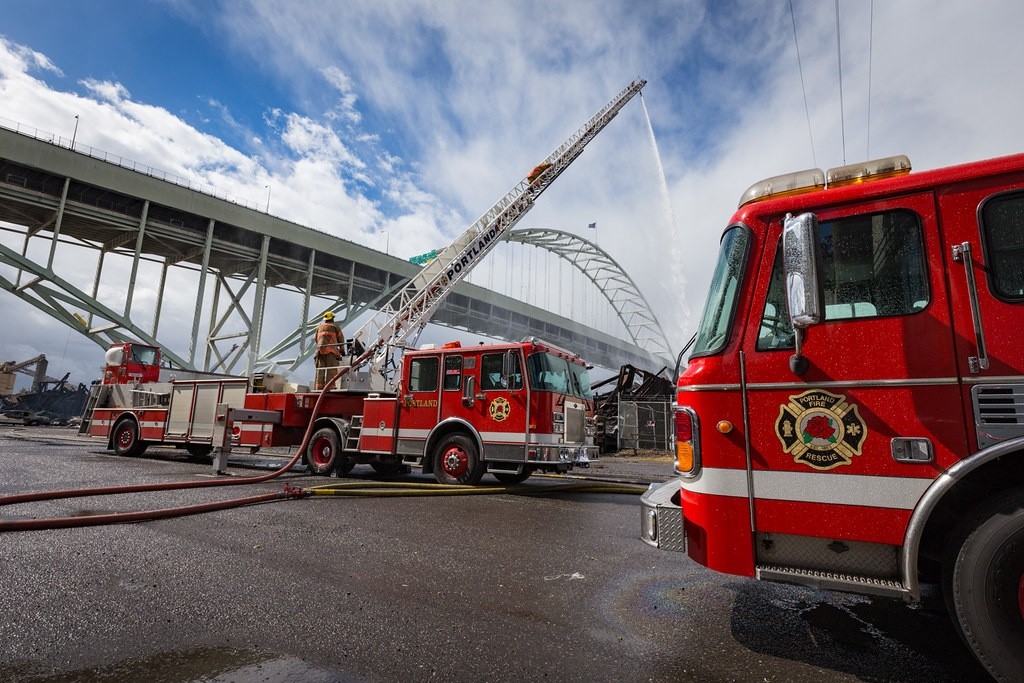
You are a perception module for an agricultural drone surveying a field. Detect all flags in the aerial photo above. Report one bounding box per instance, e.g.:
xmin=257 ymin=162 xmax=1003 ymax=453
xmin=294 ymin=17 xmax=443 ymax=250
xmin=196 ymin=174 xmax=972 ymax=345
xmin=588 ymin=223 xmax=596 ymax=228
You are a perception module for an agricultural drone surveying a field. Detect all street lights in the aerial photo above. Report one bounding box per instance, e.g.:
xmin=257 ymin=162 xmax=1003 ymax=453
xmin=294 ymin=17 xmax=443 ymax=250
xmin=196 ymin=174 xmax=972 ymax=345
xmin=265 ymin=185 xmax=271 ymax=214
xmin=521 ymin=286 xmax=528 ymax=304
xmin=381 ymin=230 xmax=389 ymax=254
xmin=71 ymin=114 xmax=79 ymax=151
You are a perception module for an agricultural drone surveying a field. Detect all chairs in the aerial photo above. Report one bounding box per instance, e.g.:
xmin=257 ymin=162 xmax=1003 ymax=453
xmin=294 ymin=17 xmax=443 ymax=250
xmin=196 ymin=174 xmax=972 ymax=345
xmin=482 ymin=376 xmax=494 ymax=390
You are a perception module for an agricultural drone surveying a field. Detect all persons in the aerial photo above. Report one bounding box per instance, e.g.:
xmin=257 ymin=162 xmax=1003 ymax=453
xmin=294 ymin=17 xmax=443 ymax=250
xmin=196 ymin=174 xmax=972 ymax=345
xmin=313 ymin=311 xmax=347 ymax=390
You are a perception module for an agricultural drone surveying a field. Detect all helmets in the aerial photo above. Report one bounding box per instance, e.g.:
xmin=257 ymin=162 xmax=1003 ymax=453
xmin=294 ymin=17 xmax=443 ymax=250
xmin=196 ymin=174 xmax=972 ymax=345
xmin=323 ymin=311 xmax=335 ymax=320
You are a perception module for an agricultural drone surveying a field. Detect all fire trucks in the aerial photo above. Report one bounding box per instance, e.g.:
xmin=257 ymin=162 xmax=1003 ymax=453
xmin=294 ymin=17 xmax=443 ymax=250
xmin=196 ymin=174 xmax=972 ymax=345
xmin=638 ymin=151 xmax=1024 ymax=683
xmin=77 ymin=76 xmax=649 ymax=486
